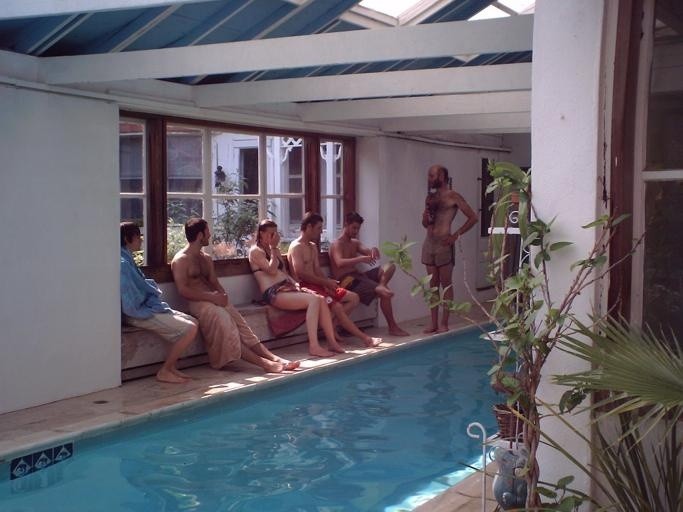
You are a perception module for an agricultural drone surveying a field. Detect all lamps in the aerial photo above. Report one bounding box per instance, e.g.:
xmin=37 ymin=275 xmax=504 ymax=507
xmin=213 ymin=164 xmax=226 ymax=191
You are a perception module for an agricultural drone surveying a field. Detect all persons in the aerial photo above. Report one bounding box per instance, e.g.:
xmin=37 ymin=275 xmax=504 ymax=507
xmin=327 ymin=211 xmax=410 ymax=336
xmin=120 ymin=222 xmax=199 ymax=383
xmin=169 ymin=217 xmax=301 ymax=373
xmin=285 ymin=211 xmax=383 ymax=347
xmin=421 ymin=165 xmax=478 ymax=335
xmin=249 ymin=219 xmax=346 ymax=358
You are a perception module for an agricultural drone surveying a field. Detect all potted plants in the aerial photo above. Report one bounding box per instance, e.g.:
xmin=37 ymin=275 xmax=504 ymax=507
xmin=378 ymin=153 xmax=665 ymax=511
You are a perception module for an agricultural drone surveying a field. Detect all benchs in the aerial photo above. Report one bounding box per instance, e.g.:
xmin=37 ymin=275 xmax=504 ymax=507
xmin=120 ymin=294 xmax=379 ymax=384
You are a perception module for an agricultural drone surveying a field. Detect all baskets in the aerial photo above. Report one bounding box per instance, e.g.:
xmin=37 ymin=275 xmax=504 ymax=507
xmin=493 ymin=403 xmax=525 ymax=443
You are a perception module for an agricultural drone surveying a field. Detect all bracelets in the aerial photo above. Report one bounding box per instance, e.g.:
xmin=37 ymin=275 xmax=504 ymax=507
xmin=269 ymin=247 xmax=277 ymax=250
